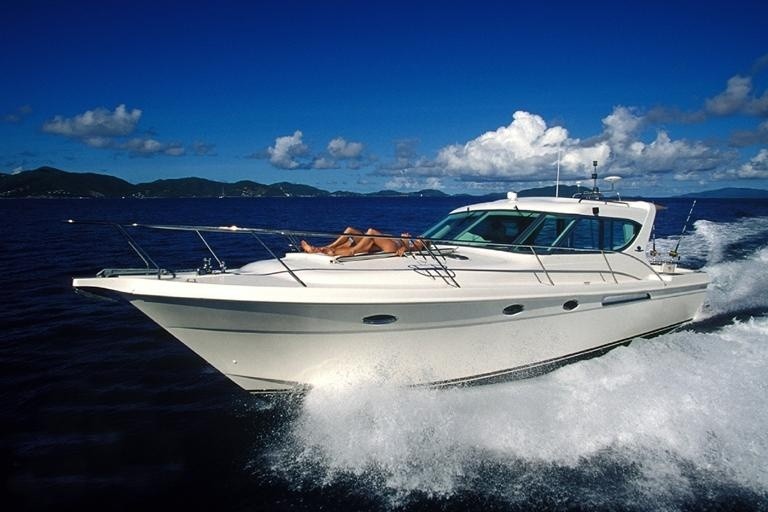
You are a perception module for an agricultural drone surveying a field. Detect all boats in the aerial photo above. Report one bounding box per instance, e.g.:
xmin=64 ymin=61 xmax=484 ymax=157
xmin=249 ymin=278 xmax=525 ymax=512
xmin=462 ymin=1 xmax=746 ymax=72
xmin=64 ymin=130 xmax=714 ymax=408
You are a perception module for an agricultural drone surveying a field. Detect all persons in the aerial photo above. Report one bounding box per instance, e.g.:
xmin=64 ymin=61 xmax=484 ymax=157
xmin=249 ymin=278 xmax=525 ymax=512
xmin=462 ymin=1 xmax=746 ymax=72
xmin=300 ymin=226 xmax=413 ymax=255
xmin=327 ymin=227 xmax=427 ymax=257
